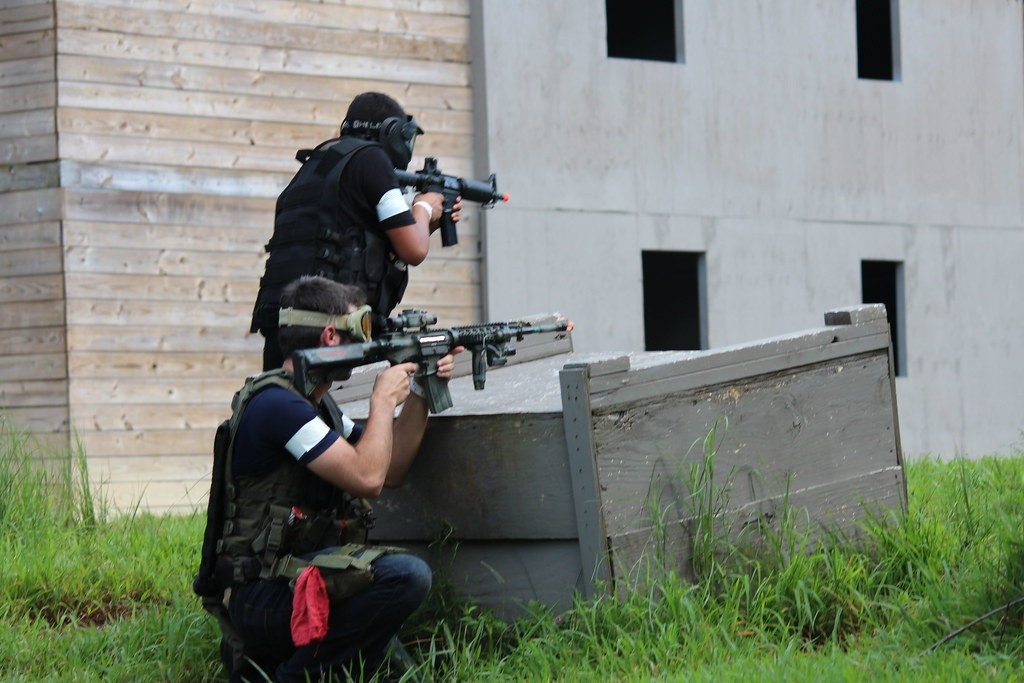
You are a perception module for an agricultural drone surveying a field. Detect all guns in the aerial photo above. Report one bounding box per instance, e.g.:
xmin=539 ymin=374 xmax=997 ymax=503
xmin=393 ymin=155 xmax=510 ymax=249
xmin=292 ymin=308 xmax=574 ymax=416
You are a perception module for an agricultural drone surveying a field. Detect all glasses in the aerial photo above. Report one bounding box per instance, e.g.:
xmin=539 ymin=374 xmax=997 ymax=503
xmin=377 ymin=114 xmax=425 ymax=171
xmin=348 ymin=305 xmax=372 ymax=342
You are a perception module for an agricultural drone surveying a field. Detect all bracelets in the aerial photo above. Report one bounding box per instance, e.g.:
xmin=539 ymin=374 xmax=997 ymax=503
xmin=413 ymin=201 xmax=432 ymax=222
xmin=409 ymin=377 xmax=428 ymax=399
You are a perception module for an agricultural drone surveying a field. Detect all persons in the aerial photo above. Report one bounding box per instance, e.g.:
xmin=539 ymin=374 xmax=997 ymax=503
xmin=192 ymin=275 xmax=466 ymax=683
xmin=247 ymin=92 xmax=464 ymax=378
